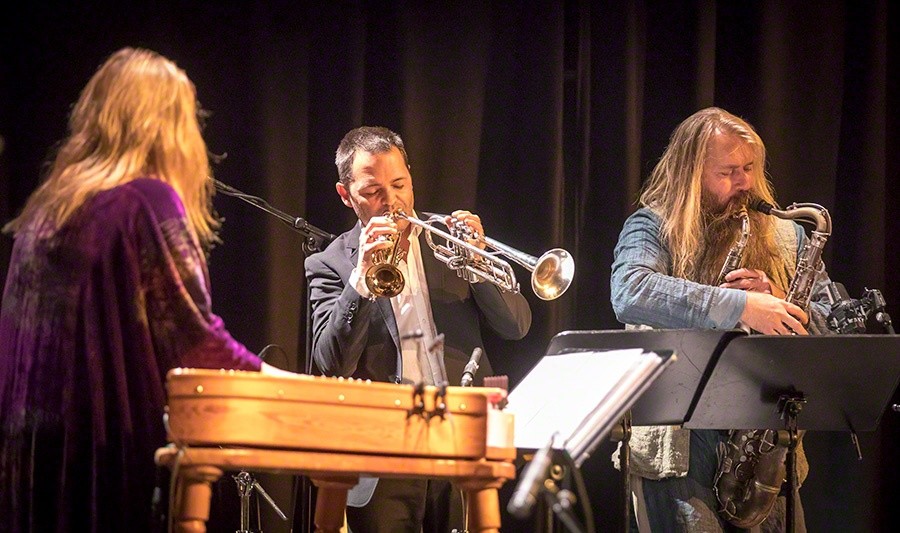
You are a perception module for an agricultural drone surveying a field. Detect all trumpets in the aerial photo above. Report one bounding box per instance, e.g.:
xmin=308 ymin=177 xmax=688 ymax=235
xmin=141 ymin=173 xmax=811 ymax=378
xmin=363 ymin=211 xmax=406 ymax=299
xmin=393 ymin=208 xmax=575 ymax=301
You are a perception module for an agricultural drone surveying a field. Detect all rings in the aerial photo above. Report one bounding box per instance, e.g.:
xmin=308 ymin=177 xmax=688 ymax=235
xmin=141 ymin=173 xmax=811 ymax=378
xmin=368 ymin=233 xmax=378 ymax=241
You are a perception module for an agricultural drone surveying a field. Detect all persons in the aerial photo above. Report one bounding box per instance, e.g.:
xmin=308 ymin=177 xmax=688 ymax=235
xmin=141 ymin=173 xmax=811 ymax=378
xmin=303 ymin=125 xmax=532 ymax=533
xmin=0 ymin=46 xmax=291 ymax=533
xmin=610 ymin=106 xmax=838 ymax=533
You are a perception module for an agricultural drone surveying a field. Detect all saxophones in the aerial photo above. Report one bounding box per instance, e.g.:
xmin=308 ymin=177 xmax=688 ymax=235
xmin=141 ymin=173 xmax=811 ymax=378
xmin=709 ymin=192 xmax=835 ymax=531
